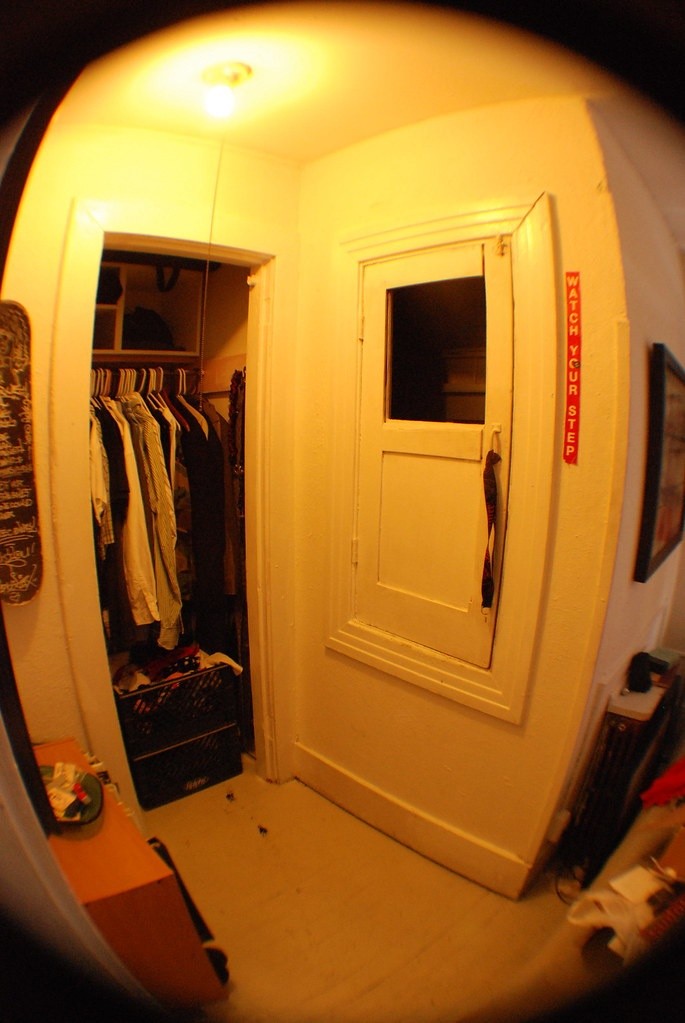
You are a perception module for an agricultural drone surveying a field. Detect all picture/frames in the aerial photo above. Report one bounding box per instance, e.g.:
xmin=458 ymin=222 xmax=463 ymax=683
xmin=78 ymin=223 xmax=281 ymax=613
xmin=633 ymin=340 xmax=685 ymax=583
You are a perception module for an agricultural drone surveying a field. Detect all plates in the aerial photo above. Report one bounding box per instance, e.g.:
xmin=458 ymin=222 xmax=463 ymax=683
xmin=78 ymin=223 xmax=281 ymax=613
xmin=40 ymin=766 xmax=103 ymax=825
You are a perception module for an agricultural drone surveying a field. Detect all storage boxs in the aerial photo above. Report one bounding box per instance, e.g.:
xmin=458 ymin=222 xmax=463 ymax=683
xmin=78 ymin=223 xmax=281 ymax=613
xmin=114 ymin=661 xmax=244 ymax=813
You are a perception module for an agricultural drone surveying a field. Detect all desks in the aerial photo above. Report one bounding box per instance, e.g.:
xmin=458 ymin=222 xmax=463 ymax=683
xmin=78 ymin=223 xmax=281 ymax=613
xmin=31 ymin=737 xmax=233 ymax=1013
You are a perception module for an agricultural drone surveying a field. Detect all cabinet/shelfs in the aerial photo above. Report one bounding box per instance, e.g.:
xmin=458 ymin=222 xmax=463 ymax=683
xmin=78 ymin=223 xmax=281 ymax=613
xmin=88 ymin=260 xmax=210 ymax=364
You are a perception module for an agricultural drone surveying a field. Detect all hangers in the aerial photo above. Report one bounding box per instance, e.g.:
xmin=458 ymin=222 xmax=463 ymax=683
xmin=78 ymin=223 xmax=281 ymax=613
xmin=92 ymin=367 xmax=192 ymax=400
